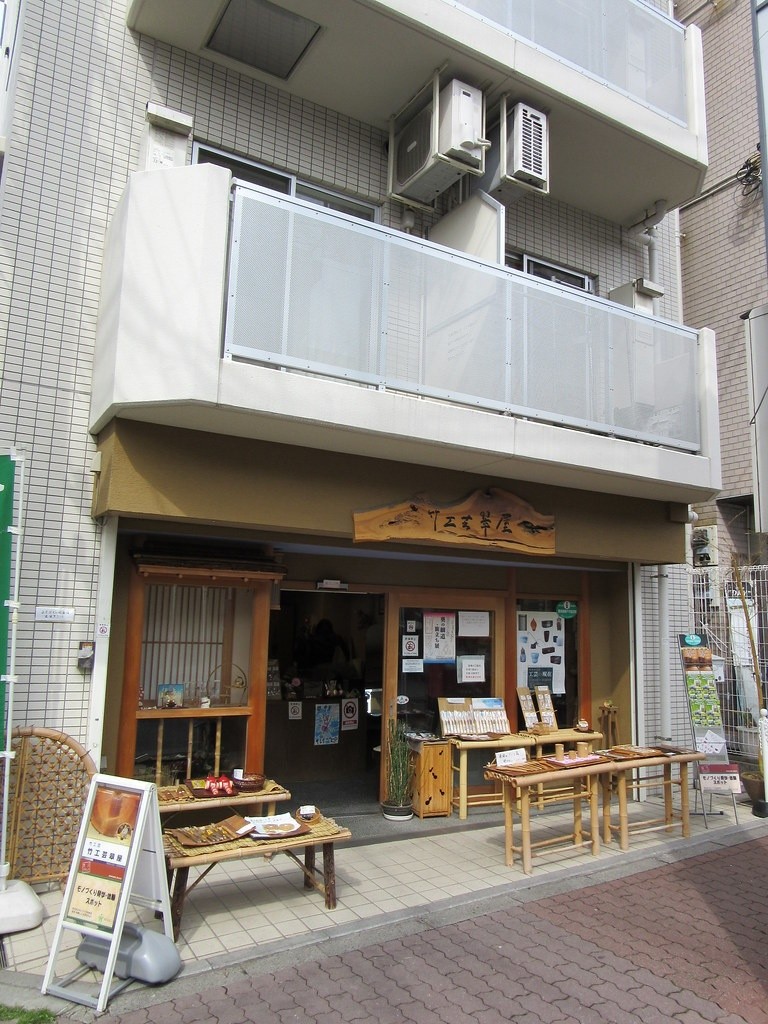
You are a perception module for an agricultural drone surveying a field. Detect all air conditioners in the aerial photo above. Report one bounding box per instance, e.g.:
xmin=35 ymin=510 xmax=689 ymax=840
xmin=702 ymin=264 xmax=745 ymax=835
xmin=385 ymin=78 xmax=486 ymax=214
xmin=458 ymin=88 xmax=552 ymax=206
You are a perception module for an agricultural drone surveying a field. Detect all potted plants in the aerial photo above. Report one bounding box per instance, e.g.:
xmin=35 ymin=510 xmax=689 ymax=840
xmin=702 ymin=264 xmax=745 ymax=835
xmin=692 ymin=530 xmax=765 ymax=801
xmin=379 ymin=718 xmax=420 ymax=821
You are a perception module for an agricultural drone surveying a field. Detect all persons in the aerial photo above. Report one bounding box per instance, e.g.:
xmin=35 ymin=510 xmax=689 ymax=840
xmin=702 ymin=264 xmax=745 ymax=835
xmin=119 ymin=825 xmax=128 ymax=839
xmin=307 ymin=619 xmax=344 ymax=664
xmin=348 ymin=619 xmax=380 ymax=668
xmin=165 ymin=719 xmax=212 ymax=769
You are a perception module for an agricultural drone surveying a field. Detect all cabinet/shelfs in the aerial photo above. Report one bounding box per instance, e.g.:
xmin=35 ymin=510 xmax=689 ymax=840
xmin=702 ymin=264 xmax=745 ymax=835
xmin=414 ymin=740 xmax=451 ymax=818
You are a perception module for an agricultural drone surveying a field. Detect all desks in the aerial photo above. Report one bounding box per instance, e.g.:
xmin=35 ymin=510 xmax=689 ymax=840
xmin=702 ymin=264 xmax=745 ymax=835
xmin=157 ymin=781 xmax=292 ymax=821
xmin=484 ymin=751 xmax=616 ymax=874
xmin=518 ymin=726 xmax=603 ymax=810
xmin=595 ymin=744 xmax=706 ymax=848
xmin=450 ymin=734 xmax=536 ymax=819
xmin=155 ymin=816 xmax=351 ymax=942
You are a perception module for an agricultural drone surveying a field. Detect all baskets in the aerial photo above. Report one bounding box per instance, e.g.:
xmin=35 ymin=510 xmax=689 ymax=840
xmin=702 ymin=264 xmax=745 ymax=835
xmin=232 ymin=772 xmax=266 ymax=793
